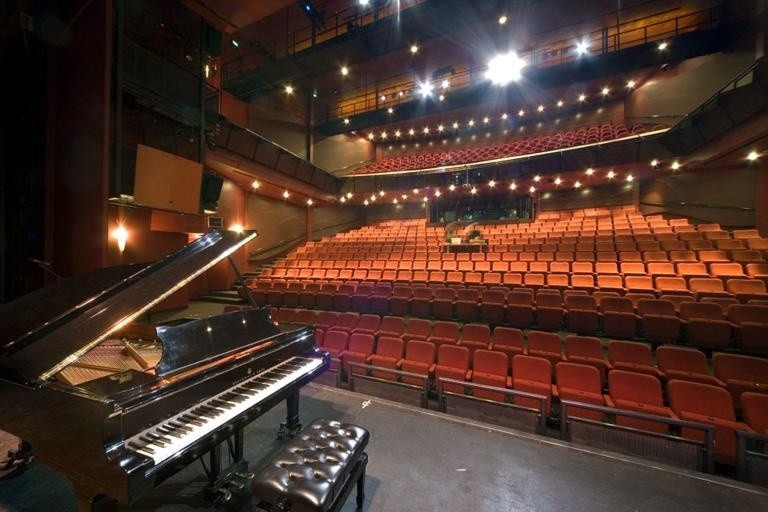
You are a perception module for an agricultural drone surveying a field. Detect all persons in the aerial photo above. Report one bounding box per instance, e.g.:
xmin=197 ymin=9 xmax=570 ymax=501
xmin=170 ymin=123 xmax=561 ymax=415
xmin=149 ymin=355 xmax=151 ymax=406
xmin=431 ymin=196 xmax=530 ymax=231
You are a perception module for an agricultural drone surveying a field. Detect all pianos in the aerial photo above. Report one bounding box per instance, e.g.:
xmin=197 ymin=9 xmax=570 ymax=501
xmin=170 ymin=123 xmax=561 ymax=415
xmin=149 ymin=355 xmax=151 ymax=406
xmin=0 ymin=229 xmax=332 ymax=512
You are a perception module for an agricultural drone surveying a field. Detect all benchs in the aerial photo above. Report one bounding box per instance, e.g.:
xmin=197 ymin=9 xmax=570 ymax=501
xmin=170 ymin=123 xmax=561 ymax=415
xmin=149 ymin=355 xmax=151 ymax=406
xmin=252 ymin=418 xmax=371 ymax=512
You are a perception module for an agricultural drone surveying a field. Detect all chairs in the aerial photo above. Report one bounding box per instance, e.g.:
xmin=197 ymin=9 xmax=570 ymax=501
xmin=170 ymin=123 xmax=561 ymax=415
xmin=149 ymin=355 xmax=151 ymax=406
xmin=348 ymin=124 xmax=671 ymax=176
xmin=487 ymin=204 xmax=767 ymax=310
xmin=240 ymin=204 xmax=443 ymax=316
xmin=224 ymin=305 xmax=765 ymax=469
xmin=443 ymin=255 xmax=486 ymax=304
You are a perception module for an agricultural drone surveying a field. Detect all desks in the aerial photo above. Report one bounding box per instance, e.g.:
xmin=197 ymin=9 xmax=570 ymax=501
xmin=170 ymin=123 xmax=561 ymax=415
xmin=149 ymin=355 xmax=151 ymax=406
xmin=441 ymin=240 xmax=488 ymax=254
